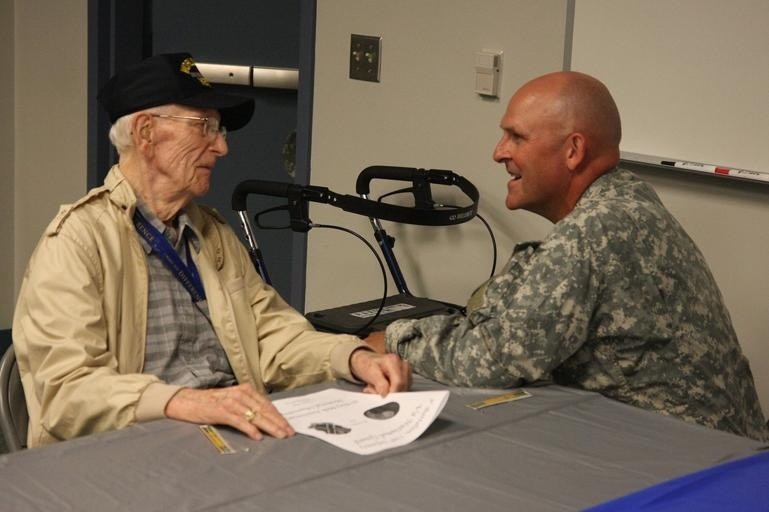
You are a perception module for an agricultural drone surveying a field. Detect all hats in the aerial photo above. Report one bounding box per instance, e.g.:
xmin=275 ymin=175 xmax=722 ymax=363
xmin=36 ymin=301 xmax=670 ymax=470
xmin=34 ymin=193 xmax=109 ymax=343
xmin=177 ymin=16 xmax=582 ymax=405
xmin=97 ymin=51 xmax=256 ymax=133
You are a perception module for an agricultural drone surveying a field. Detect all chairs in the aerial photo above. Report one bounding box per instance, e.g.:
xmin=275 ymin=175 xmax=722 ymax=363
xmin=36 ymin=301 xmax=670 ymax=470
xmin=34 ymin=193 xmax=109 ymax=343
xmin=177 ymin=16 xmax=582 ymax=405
xmin=0 ymin=343 xmax=28 ymax=453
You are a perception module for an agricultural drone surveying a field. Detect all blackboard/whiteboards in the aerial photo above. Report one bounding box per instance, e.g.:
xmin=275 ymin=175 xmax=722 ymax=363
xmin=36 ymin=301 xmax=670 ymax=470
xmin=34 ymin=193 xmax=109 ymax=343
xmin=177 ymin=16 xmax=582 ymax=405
xmin=562 ymin=0 xmax=769 ymax=185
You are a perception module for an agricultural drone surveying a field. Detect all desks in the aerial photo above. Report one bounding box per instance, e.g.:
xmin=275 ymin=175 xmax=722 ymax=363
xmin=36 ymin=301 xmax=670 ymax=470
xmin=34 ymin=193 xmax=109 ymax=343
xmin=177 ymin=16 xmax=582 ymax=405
xmin=0 ymin=372 xmax=769 ymax=512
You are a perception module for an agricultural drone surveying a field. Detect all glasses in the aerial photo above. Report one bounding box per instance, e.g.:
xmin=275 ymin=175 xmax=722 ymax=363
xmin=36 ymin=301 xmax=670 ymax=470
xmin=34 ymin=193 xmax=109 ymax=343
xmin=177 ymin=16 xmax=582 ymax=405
xmin=152 ymin=113 xmax=228 ymax=142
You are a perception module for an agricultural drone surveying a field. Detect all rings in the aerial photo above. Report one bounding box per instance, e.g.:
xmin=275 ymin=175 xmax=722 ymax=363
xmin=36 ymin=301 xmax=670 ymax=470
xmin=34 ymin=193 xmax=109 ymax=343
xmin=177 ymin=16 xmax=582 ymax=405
xmin=243 ymin=408 xmax=257 ymax=422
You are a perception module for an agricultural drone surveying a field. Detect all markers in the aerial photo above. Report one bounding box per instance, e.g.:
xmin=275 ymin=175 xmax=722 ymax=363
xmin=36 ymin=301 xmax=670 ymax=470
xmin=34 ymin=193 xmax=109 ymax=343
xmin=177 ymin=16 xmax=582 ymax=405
xmin=661 ymin=161 xmax=769 ymax=182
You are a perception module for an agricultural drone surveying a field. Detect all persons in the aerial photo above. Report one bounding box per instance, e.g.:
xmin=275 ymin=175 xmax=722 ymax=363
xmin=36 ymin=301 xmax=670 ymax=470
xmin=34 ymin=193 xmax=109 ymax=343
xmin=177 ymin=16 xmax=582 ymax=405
xmin=13 ymin=55 xmax=414 ymax=451
xmin=360 ymin=70 xmax=769 ymax=443
